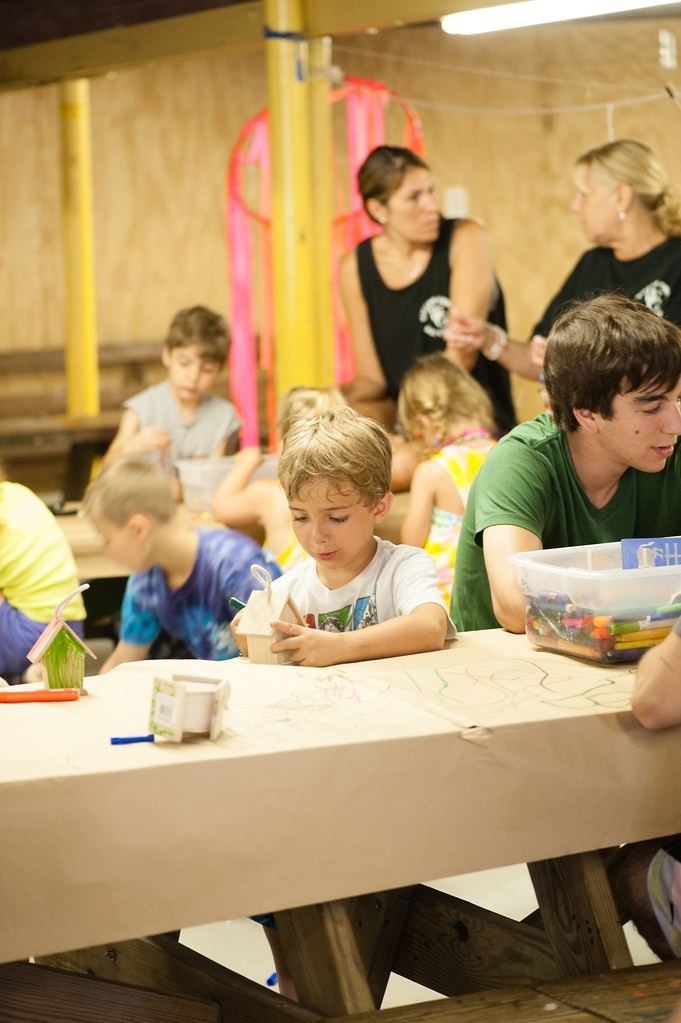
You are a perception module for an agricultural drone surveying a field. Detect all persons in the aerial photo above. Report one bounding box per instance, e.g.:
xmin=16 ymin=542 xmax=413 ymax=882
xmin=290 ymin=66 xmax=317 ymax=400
xmin=101 ymin=306 xmax=242 ymax=471
xmin=284 ymin=145 xmax=517 ymax=440
xmin=450 ymin=288 xmax=680 ymax=966
xmin=78 ymin=455 xmax=283 ymax=676
xmin=399 ymin=351 xmax=500 ymax=613
xmin=442 ymin=142 xmax=681 ymax=416
xmin=211 ymin=385 xmax=417 ymax=577
xmin=1 ymin=482 xmax=86 ymax=688
xmin=232 ymin=404 xmax=458 ymax=667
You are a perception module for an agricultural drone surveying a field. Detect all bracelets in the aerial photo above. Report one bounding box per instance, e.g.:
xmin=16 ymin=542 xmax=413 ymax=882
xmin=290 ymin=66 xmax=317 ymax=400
xmin=484 ymin=324 xmax=507 ymax=360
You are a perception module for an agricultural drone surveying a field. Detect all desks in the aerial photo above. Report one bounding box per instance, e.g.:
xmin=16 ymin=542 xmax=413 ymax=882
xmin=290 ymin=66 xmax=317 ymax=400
xmin=0 ymin=627 xmax=681 ymax=1023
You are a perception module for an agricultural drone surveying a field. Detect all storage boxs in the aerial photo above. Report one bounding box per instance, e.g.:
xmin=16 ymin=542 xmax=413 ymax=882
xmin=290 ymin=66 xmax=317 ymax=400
xmin=510 ymin=537 xmax=681 ymax=664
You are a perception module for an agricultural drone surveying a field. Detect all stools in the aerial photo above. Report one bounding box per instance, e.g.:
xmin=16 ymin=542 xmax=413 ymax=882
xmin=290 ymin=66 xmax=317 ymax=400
xmin=11 ymin=637 xmax=115 ymax=684
